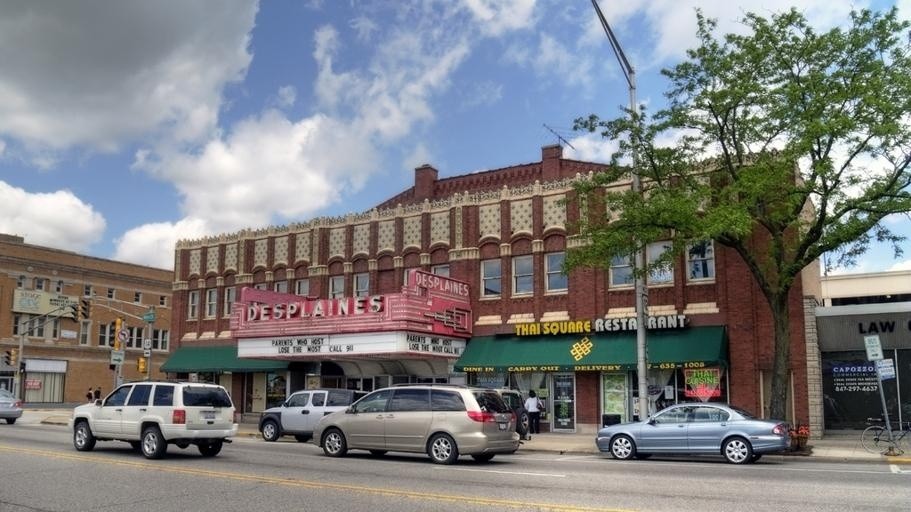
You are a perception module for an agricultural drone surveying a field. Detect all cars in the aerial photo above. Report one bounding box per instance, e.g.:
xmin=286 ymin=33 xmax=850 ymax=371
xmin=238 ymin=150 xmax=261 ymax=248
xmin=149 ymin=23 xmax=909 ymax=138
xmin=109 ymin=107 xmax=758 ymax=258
xmin=596 ymin=401 xmax=796 ymax=466
xmin=0 ymin=387 xmax=24 ymax=424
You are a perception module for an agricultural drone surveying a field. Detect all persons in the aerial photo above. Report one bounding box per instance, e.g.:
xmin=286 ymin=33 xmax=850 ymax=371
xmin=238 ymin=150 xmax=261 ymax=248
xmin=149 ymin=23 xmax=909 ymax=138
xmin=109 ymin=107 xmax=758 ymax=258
xmin=525 ymin=390 xmax=544 ymax=433
xmin=85 ymin=386 xmax=93 ymax=403
xmin=94 ymin=386 xmax=104 ymax=403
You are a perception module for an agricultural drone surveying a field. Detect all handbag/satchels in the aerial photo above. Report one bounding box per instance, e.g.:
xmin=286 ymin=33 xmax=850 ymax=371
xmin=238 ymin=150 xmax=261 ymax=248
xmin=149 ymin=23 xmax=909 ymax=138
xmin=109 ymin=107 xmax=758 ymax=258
xmin=536 ymin=401 xmax=545 ymax=410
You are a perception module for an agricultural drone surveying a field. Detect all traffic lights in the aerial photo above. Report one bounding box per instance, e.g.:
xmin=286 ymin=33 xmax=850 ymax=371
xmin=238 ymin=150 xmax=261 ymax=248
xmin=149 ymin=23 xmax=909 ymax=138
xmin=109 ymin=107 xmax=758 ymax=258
xmin=4 ymin=349 xmax=16 ymax=366
xmin=71 ymin=302 xmax=78 ymax=321
xmin=136 ymin=357 xmax=148 ymax=372
xmin=82 ymin=299 xmax=90 ymax=317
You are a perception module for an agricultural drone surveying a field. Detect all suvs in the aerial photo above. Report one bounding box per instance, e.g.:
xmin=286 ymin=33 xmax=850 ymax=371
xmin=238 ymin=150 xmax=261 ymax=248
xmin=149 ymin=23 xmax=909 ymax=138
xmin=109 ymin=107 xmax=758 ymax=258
xmin=69 ymin=379 xmax=241 ymax=460
xmin=481 ymin=383 xmax=531 ymax=438
xmin=258 ymin=386 xmax=369 ymax=441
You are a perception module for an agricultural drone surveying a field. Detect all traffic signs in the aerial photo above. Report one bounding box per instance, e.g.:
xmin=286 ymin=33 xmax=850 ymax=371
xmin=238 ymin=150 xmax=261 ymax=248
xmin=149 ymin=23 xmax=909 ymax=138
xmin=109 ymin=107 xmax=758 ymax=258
xmin=864 ymin=335 xmax=883 ymax=360
xmin=142 ymin=312 xmax=156 ymax=322
xmin=108 ymin=350 xmax=125 ymax=366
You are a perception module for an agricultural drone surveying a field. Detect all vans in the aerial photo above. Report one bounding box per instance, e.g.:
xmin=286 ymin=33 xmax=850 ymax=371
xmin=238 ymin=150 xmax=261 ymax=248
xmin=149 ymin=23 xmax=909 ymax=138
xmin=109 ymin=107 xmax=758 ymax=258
xmin=314 ymin=382 xmax=522 ymax=466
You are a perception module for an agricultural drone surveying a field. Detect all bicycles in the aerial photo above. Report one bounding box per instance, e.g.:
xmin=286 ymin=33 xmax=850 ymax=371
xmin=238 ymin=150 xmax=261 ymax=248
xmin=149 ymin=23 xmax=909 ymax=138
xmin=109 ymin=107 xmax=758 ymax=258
xmin=862 ymin=413 xmax=911 ymax=453
